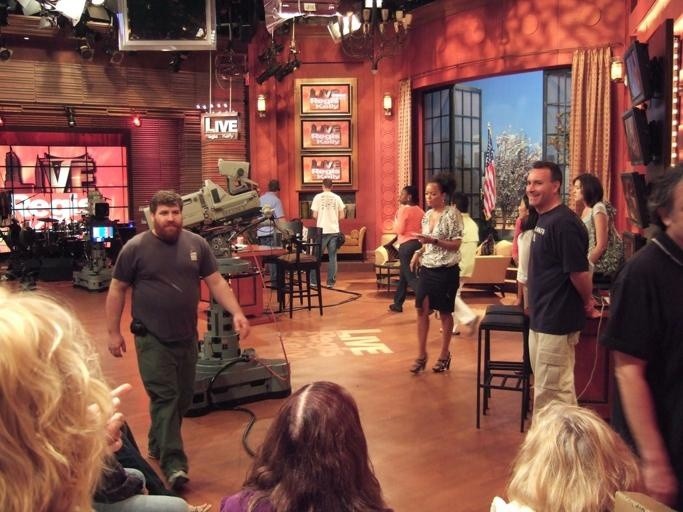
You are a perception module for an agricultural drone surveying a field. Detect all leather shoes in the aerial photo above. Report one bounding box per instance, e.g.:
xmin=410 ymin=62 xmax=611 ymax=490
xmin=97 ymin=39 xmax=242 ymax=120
xmin=390 ymin=304 xmax=402 ymax=312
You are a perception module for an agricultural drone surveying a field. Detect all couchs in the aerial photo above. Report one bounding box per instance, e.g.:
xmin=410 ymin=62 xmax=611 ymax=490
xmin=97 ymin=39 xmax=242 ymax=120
xmin=322 ymin=227 xmax=367 ymax=260
xmin=375 ymin=234 xmax=415 ymax=294
xmin=458 ymin=238 xmax=514 ymax=297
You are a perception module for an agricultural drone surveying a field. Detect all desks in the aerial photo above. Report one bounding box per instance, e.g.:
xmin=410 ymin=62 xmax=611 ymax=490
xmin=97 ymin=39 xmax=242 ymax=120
xmin=574 ymin=285 xmax=614 ymax=424
xmin=373 ymin=259 xmax=401 ymax=292
xmin=66 ymin=226 xmax=136 ymax=263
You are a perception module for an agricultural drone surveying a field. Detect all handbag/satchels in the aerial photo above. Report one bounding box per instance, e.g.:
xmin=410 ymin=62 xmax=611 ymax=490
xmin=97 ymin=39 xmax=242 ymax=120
xmin=337 ymin=233 xmax=345 ymax=248
xmin=589 ymin=203 xmax=625 ymax=282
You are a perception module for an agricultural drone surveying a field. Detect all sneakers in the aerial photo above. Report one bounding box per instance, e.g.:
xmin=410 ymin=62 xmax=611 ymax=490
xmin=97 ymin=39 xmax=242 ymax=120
xmin=168 ymin=470 xmax=188 ymax=489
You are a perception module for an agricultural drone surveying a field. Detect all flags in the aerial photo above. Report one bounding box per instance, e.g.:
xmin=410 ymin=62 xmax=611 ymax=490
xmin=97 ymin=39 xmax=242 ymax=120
xmin=483 ymin=130 xmax=496 ymax=220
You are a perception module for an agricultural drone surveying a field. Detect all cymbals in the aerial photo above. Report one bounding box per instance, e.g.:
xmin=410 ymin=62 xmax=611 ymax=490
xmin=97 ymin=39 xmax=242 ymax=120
xmin=37 ymin=217 xmax=59 ymax=222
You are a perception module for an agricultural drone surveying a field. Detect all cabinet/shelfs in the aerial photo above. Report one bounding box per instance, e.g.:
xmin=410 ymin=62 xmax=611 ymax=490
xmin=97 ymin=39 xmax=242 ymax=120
xmin=197 ymin=263 xmax=282 ymax=327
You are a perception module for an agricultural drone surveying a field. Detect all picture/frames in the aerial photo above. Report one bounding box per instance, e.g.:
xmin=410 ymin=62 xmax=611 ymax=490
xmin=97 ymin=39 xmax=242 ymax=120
xmin=300 ymin=82 xmax=353 ymax=187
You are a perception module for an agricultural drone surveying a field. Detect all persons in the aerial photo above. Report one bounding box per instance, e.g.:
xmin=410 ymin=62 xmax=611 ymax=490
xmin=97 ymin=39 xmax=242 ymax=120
xmin=105 ymin=189 xmax=250 ymax=490
xmin=0 ymin=280 xmax=131 ymax=512
xmin=218 ymin=381 xmax=395 ymax=512
xmin=380 ymin=177 xmax=480 ymax=373
xmin=599 ymin=161 xmax=683 ymax=512
xmin=256 ymin=179 xmax=345 ymax=288
xmin=489 ymin=399 xmax=641 ymax=512
xmin=516 ymin=195 xmax=539 ymax=285
xmin=573 ymin=172 xmax=625 ymax=283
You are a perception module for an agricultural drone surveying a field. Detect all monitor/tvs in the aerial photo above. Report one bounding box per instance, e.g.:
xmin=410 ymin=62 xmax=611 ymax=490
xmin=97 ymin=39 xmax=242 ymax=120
xmin=621 ymin=171 xmax=650 ymax=227
xmin=619 ymin=108 xmax=650 ymax=167
xmin=623 ymin=39 xmax=651 ymax=106
xmin=116 ymin=0 xmax=218 ymax=52
xmin=91 ymin=226 xmax=116 ymax=243
xmin=622 ymin=232 xmax=644 ymax=266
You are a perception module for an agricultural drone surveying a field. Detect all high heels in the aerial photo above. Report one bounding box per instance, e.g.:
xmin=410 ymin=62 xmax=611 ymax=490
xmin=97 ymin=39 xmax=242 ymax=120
xmin=410 ymin=356 xmax=425 ymax=371
xmin=432 ymin=352 xmax=450 ymax=371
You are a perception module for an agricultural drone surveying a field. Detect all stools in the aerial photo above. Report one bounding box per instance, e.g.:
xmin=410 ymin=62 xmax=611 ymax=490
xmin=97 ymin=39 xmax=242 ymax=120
xmin=485 ymin=303 xmax=532 ymax=410
xmin=476 ymin=314 xmax=530 ymax=433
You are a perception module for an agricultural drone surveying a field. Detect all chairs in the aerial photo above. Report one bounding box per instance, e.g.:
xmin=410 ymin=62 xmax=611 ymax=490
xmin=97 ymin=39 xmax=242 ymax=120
xmin=278 ymin=218 xmax=303 ymax=309
xmin=275 ymin=226 xmax=323 ymax=319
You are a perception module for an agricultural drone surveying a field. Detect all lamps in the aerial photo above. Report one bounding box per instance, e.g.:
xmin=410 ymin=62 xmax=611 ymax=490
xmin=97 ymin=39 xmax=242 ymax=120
xmin=610 ymin=59 xmax=628 ymax=87
xmin=256 ymin=94 xmax=267 ymax=119
xmin=383 ymin=96 xmax=392 ymax=116
xmin=327 ymin=0 xmax=413 ymax=74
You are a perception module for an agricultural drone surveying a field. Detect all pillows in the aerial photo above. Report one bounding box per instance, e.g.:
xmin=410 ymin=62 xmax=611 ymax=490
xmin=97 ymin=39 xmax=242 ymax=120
xmin=475 ymin=233 xmax=495 ymax=255
xmin=351 ymin=229 xmax=359 ymax=238
